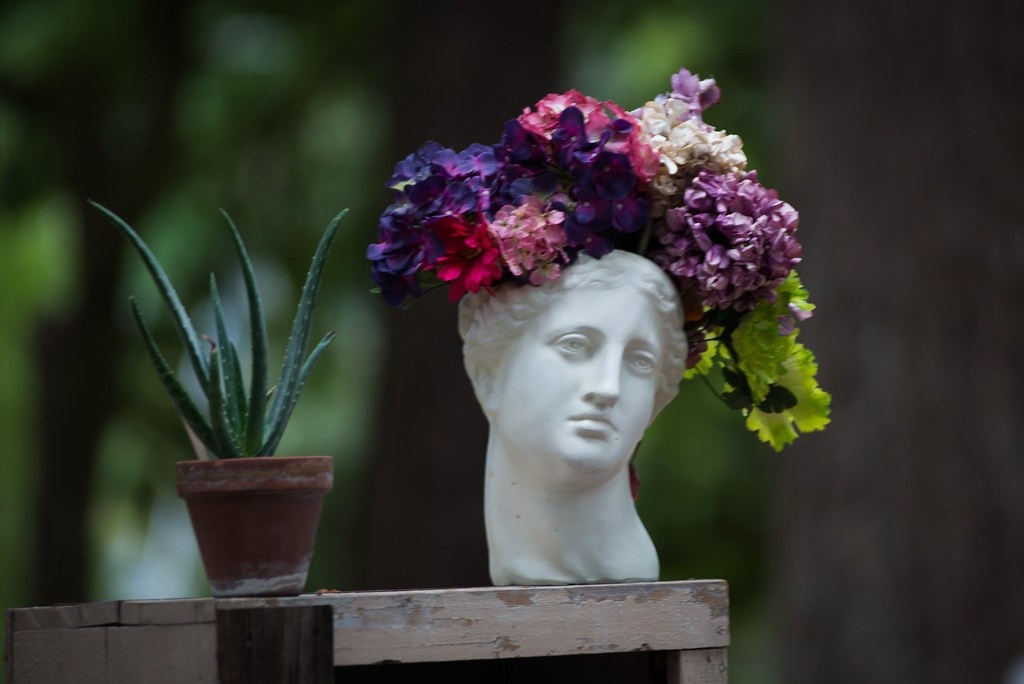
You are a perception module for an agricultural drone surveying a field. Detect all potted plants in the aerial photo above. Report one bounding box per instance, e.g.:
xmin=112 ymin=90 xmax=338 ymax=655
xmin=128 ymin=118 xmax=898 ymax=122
xmin=85 ymin=196 xmax=350 ymax=596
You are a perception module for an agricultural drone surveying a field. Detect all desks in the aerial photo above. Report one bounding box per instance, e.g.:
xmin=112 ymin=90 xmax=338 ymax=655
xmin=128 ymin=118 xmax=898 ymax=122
xmin=0 ymin=578 xmax=731 ymax=684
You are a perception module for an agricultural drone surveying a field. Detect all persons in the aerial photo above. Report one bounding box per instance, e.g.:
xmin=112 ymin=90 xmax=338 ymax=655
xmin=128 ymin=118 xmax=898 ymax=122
xmin=457 ymin=248 xmax=691 ymax=586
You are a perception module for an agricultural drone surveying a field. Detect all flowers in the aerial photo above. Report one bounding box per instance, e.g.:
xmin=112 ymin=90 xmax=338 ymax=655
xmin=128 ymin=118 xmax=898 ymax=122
xmin=371 ymin=69 xmax=832 ymax=453
